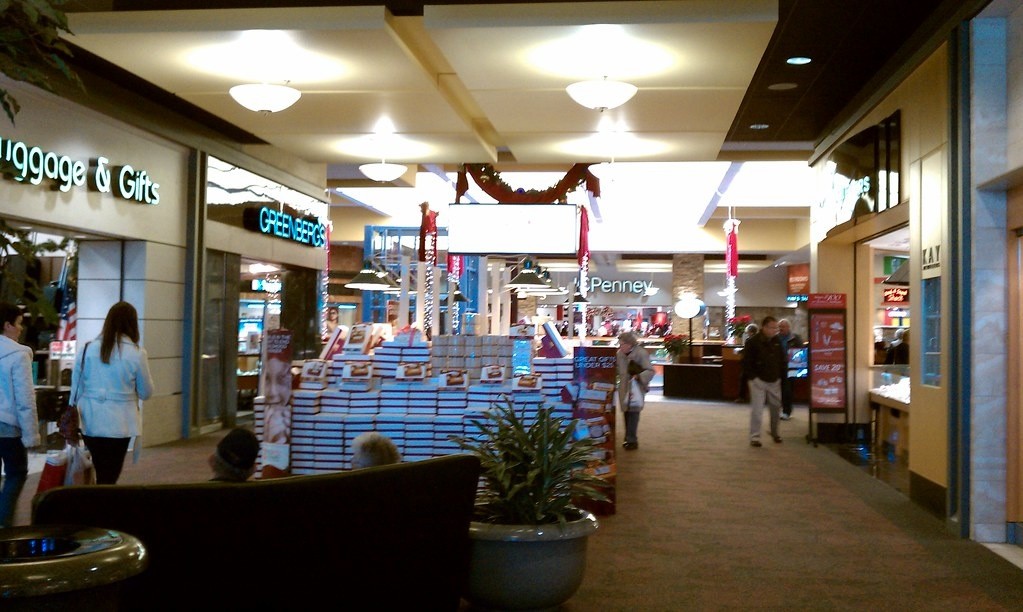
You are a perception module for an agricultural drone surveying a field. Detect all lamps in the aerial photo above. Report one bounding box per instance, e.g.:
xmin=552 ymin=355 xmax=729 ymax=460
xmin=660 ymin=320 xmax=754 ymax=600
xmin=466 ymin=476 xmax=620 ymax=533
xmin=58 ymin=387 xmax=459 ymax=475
xmin=505 ymin=253 xmax=591 ymax=305
xmin=344 ymin=250 xmax=471 ymax=303
xmin=564 ymin=79 xmax=639 ymax=114
xmin=359 ymin=164 xmax=408 ymax=184
xmin=230 ymin=84 xmax=300 ymax=115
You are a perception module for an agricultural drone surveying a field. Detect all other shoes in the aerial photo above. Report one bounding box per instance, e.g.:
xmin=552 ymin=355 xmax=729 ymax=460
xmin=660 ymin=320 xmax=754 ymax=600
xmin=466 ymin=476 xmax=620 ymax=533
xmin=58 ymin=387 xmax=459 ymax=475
xmin=751 ymin=440 xmax=761 ymax=446
xmin=623 ymin=442 xmax=637 ymax=449
xmin=772 ymin=433 xmax=782 ymax=442
xmin=780 ymin=413 xmax=789 ymax=419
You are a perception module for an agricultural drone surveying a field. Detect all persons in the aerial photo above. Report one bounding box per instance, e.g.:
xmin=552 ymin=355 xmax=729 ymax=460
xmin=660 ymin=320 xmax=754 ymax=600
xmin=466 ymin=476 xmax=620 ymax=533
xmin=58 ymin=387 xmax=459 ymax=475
xmin=0 ymin=301 xmax=41 ymax=526
xmin=775 ymin=318 xmax=802 ymax=420
xmin=264 ymin=353 xmax=290 ymax=443
xmin=68 ymin=301 xmax=155 ymax=486
xmin=615 ymin=332 xmax=655 ymax=448
xmin=327 ymin=308 xmax=338 ymax=334
xmin=207 ymin=427 xmax=260 ymax=482
xmin=734 ymin=324 xmax=757 ymax=404
xmin=743 ymin=316 xmax=787 ymax=447
xmin=885 ymin=328 xmax=910 ymax=365
xmin=351 ymin=431 xmax=401 ymax=470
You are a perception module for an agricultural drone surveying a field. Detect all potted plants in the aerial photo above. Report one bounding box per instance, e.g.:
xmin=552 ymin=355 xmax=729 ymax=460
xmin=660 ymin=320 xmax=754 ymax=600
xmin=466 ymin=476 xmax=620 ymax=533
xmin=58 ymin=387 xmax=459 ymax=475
xmin=447 ymin=398 xmax=619 ymax=609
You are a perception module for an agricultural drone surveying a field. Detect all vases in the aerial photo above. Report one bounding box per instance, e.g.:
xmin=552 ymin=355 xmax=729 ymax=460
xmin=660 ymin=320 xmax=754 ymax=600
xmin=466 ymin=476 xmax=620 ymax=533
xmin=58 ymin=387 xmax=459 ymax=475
xmin=734 ymin=337 xmax=743 ymax=344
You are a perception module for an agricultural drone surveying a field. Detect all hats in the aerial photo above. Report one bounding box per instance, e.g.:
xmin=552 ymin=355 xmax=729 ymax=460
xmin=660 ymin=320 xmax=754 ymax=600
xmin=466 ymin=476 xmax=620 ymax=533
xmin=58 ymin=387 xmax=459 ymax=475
xmin=218 ymin=429 xmax=260 ymax=470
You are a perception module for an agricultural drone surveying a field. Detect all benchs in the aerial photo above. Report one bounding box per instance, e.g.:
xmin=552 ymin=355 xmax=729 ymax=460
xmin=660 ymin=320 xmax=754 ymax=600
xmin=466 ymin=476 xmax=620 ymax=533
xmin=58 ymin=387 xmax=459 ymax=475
xmin=31 ymin=456 xmax=480 ymax=611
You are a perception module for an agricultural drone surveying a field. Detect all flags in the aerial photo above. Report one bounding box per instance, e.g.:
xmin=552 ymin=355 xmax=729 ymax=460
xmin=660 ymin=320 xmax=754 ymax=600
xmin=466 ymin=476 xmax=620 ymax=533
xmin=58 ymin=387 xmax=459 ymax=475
xmin=56 ymin=283 xmax=76 ymax=340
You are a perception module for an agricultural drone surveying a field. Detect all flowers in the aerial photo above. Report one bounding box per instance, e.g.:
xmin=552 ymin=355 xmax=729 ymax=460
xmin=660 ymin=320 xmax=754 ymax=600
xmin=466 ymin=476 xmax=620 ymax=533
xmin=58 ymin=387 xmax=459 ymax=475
xmin=662 ymin=332 xmax=693 ymax=356
xmin=725 ymin=315 xmax=751 ymax=338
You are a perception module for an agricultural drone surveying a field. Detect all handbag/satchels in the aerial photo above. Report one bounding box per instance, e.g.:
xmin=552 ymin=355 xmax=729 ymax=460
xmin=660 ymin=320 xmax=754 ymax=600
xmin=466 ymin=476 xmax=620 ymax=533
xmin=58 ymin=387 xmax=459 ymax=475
xmin=627 ymin=360 xmax=644 ymax=376
xmin=64 ymin=446 xmax=96 ymax=486
xmin=36 ymin=447 xmax=73 ymax=494
xmin=622 ymin=378 xmax=643 ymax=407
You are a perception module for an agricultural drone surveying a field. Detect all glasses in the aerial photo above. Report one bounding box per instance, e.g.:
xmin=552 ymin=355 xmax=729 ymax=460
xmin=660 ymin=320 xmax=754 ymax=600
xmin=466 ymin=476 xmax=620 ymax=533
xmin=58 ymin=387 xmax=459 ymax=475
xmin=619 ymin=342 xmax=626 ymax=348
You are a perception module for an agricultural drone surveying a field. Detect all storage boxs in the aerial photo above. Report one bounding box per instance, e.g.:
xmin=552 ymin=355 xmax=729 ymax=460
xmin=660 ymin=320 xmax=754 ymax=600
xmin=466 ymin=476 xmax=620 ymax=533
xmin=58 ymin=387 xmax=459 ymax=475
xmin=253 ymin=322 xmax=574 ymax=506
